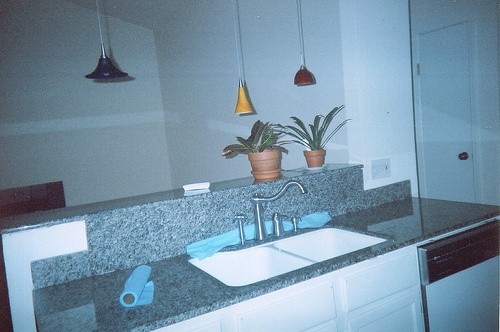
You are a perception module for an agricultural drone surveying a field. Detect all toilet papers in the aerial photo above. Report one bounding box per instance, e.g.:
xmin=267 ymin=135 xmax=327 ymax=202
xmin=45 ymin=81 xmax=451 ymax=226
xmin=119 ymin=264 xmax=155 ymax=308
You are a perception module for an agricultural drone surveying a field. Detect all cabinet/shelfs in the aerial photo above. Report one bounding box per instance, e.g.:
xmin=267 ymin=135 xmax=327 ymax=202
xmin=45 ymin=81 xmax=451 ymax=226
xmin=417 ymin=219 xmax=500 ymax=332
xmin=156 ymin=246 xmax=426 ymax=332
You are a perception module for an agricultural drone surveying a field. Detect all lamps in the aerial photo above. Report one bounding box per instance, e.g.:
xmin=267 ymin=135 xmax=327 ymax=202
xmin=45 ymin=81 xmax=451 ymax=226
xmin=235 ymin=0 xmax=253 ymax=114
xmin=85 ymin=0 xmax=128 ymax=79
xmin=294 ymin=0 xmax=316 ymax=85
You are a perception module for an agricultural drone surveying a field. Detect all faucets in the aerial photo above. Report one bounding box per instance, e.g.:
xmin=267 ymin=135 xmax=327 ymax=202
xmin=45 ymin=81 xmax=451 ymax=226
xmin=252 ymin=180 xmax=308 ymax=242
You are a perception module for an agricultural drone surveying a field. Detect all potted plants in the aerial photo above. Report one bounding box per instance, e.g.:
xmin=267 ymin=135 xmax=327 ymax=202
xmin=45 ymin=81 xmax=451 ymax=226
xmin=283 ymin=104 xmax=352 ymax=170
xmin=222 ymin=119 xmax=290 ymax=179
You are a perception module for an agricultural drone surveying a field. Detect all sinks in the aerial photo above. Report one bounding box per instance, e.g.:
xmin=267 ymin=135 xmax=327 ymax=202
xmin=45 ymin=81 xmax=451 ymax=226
xmin=186 ymin=241 xmax=317 ymax=288
xmin=273 ymin=226 xmax=389 ymax=263
